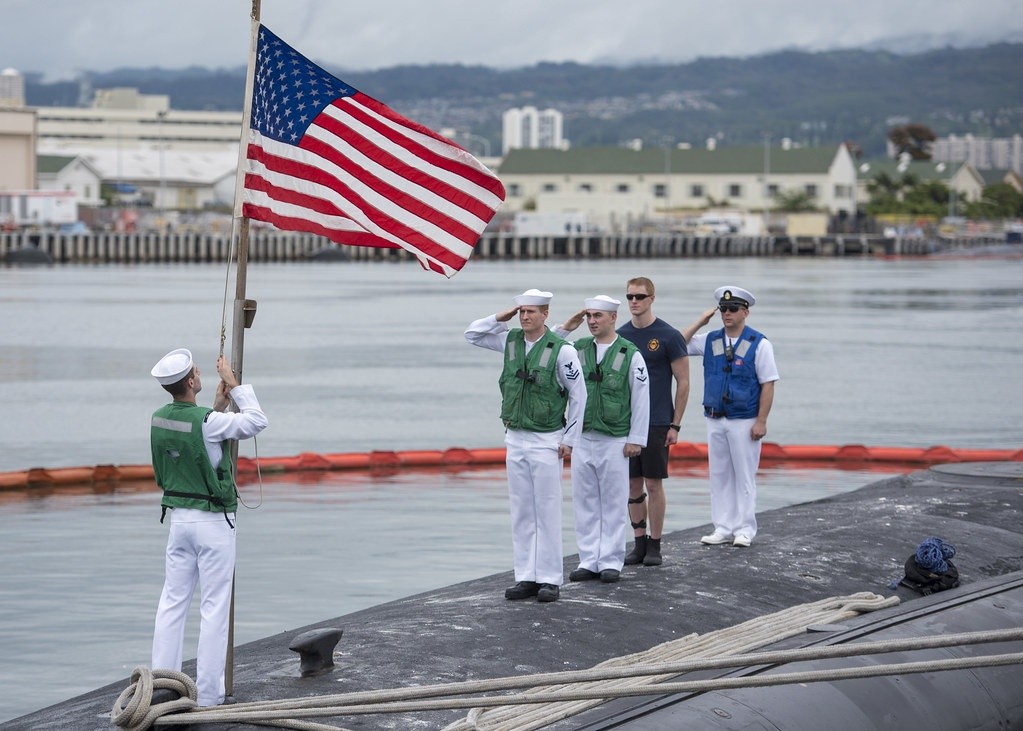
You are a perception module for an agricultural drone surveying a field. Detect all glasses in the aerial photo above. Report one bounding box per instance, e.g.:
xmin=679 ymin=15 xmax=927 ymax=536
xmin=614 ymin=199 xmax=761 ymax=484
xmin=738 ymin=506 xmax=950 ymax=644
xmin=626 ymin=294 xmax=652 ymax=300
xmin=720 ymin=306 xmax=746 ymax=312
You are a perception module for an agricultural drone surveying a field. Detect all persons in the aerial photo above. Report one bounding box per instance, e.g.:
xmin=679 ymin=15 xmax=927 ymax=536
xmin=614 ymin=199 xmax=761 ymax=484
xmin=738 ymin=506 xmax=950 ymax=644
xmin=463 ymin=288 xmax=587 ymax=602
xmin=151 ymin=349 xmax=268 ymax=709
xmin=548 ymin=294 xmax=651 ymax=581
xmin=685 ymin=285 xmax=780 ymax=548
xmin=615 ymin=276 xmax=690 ymax=566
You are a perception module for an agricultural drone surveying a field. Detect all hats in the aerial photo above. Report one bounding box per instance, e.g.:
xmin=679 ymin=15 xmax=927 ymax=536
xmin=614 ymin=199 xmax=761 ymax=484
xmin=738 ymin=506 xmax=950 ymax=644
xmin=513 ymin=288 xmax=553 ymax=306
xmin=714 ymin=286 xmax=755 ymax=309
xmin=151 ymin=348 xmax=192 ymax=385
xmin=584 ymin=295 xmax=620 ymax=311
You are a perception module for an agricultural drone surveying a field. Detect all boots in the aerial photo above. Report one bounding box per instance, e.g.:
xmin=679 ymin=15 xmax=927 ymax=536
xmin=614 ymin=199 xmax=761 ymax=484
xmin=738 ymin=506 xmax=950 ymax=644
xmin=625 ymin=537 xmax=648 ymax=564
xmin=644 ymin=540 xmax=662 ymax=566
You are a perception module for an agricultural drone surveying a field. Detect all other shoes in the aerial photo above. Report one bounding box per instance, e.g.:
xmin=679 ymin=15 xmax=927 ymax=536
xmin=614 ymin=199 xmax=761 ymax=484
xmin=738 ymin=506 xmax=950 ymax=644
xmin=217 ymin=697 xmax=236 ymax=705
xmin=569 ymin=568 xmax=598 ymax=581
xmin=600 ymin=569 xmax=620 ymax=582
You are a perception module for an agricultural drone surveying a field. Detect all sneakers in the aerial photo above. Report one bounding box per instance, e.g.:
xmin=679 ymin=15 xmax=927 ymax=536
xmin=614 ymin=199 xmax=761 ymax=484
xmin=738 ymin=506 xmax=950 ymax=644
xmin=701 ymin=532 xmax=733 ymax=544
xmin=505 ymin=581 xmax=539 ymax=598
xmin=538 ymin=582 xmax=560 ymax=602
xmin=733 ymin=535 xmax=751 ymax=546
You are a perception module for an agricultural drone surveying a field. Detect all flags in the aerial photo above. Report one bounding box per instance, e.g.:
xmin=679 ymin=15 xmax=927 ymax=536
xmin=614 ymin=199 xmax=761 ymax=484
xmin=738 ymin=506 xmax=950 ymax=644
xmin=232 ymin=19 xmax=506 ymax=281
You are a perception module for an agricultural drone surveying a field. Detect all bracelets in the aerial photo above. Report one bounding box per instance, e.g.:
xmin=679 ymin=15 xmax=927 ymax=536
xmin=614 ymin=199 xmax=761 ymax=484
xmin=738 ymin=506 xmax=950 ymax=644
xmin=669 ymin=423 xmax=681 ymax=432
